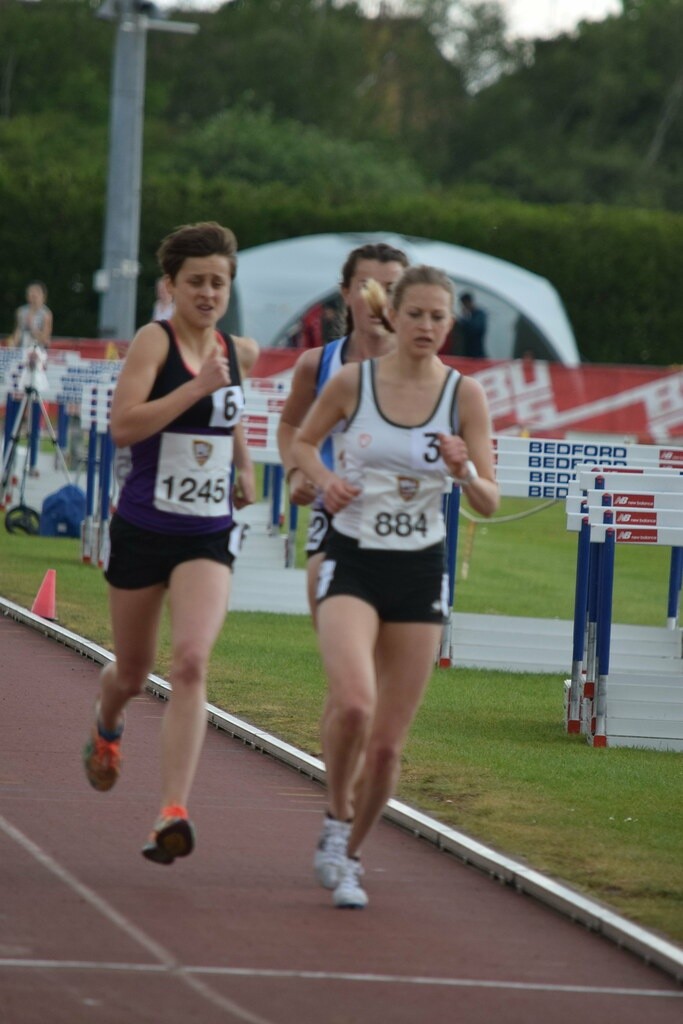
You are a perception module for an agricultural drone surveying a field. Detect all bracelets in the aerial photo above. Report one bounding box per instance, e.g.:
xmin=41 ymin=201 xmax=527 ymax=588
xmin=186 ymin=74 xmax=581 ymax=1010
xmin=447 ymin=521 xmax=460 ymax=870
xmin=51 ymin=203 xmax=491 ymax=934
xmin=450 ymin=460 xmax=477 ymax=486
xmin=286 ymin=467 xmax=298 ymax=481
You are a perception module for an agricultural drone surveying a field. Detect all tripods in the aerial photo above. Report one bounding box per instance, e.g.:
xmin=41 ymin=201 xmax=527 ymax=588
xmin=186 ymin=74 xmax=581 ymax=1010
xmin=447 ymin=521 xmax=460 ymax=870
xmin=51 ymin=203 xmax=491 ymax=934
xmin=0 ymin=349 xmax=72 ymax=508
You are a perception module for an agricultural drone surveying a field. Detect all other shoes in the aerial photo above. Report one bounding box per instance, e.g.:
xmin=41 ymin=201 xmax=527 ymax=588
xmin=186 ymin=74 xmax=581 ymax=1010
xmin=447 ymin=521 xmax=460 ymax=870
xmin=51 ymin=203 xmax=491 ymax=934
xmin=83 ymin=703 xmax=124 ymax=792
xmin=313 ymin=835 xmax=345 ymax=890
xmin=331 ymin=865 xmax=368 ymax=908
xmin=140 ymin=812 xmax=195 ymax=866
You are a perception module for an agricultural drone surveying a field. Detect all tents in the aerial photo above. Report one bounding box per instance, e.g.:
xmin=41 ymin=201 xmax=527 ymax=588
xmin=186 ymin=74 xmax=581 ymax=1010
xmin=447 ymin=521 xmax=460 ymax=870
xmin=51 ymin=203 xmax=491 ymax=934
xmin=216 ymin=231 xmax=581 ymax=367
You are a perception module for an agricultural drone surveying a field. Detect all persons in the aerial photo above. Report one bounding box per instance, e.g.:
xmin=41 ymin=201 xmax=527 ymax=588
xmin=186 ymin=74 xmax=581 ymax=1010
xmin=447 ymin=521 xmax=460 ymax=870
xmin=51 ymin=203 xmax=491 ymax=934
xmin=300 ymin=296 xmax=341 ymax=348
xmin=456 ymin=292 xmax=488 ymax=359
xmin=152 ymin=277 xmax=175 ymax=320
xmin=4 ymin=280 xmax=53 ymax=476
xmin=286 ymin=265 xmax=501 ymax=909
xmin=79 ymin=221 xmax=257 ymax=865
xmin=275 ymin=243 xmax=411 ymax=751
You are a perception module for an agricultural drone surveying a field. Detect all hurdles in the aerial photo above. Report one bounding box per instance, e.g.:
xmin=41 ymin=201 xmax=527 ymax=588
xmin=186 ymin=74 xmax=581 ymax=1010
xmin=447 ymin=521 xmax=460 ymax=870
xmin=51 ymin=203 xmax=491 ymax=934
xmin=435 ymin=429 xmax=682 ymax=675
xmin=0 ymin=346 xmax=304 ymax=572
xmin=223 ymin=409 xmax=312 ymax=616
xmin=561 ymin=464 xmax=683 ymax=753
